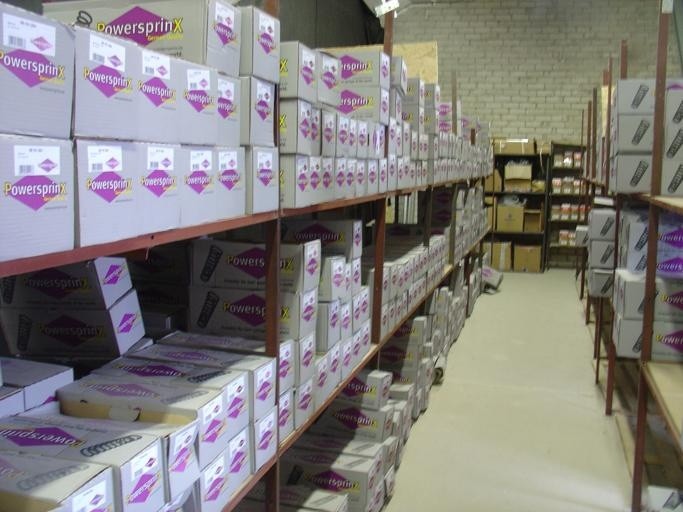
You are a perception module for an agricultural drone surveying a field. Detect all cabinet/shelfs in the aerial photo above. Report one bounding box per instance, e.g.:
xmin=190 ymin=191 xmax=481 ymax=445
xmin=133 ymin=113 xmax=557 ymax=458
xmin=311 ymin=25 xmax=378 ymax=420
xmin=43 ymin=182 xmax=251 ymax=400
xmin=488 ymin=139 xmax=587 ymax=272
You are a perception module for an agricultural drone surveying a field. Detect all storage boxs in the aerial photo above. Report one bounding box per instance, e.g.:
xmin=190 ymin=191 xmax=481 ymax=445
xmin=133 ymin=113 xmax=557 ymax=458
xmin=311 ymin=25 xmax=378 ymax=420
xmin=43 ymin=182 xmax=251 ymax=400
xmin=0 ymin=448 xmax=119 ymax=512
xmin=12 ymin=338 xmax=152 ymax=374
xmin=138 ymin=142 xmax=180 ymax=235
xmin=199 ymin=449 xmax=231 ymax=512
xmin=0 ymin=255 xmax=132 ymax=311
xmin=0 ymin=357 xmax=74 ymax=412
xmin=0 ymin=289 xmax=146 ymax=358
xmin=185 ymin=286 xmax=318 ymax=340
xmin=188 ymin=234 xmax=320 ymax=297
xmin=280 ymin=37 xmax=485 ymax=210
xmin=137 ymin=303 xmax=183 ymax=340
xmin=251 ymin=405 xmax=278 ymax=472
xmin=523 ymin=209 xmax=544 ymax=234
xmin=129 ymin=342 xmax=277 ymax=419
xmin=581 ymin=79 xmax=683 ymax=193
xmin=0 ymin=384 xmax=25 ymax=416
xmin=574 ymin=197 xmax=683 ymax=362
xmin=132 ymin=281 xmax=191 ymax=310
xmin=496 ymin=204 xmax=524 ymax=233
xmin=134 ymin=44 xmax=178 ymax=144
xmin=21 ymin=400 xmax=201 ymax=499
xmin=175 ymin=60 xmax=218 ymax=148
xmin=74 ymin=138 xmax=138 ymax=247
xmin=0 ymin=1 xmax=76 ymax=140
xmin=531 ymin=180 xmax=545 ymax=192
xmin=218 ymin=72 xmax=240 ymax=149
xmin=72 ymin=25 xmax=136 ymax=141
xmin=0 ymin=416 xmax=167 ymax=512
xmin=493 ymin=240 xmax=514 ymax=274
xmin=513 ymin=243 xmax=542 ymax=274
xmin=53 ymin=372 xmax=227 ymax=467
xmin=127 ymin=244 xmax=194 ymax=288
xmin=0 ymin=133 xmax=75 ymax=263
xmin=226 ymin=424 xmax=253 ymax=495
xmin=246 ymin=147 xmax=279 ymax=215
xmin=485 ymin=169 xmax=502 ymax=192
xmin=236 ymin=5 xmax=280 ymax=85
xmin=41 ymin=0 xmax=243 ymax=76
xmin=486 ymin=138 xmax=552 ymax=156
xmin=178 ymin=147 xmax=216 ymax=230
xmin=211 ymin=146 xmax=247 ymax=221
xmin=239 ymin=75 xmax=276 ymax=147
xmin=503 ymin=164 xmax=532 ymax=193
xmin=159 ymin=329 xmax=295 ymax=395
xmin=99 ymin=354 xmax=249 ymax=441
